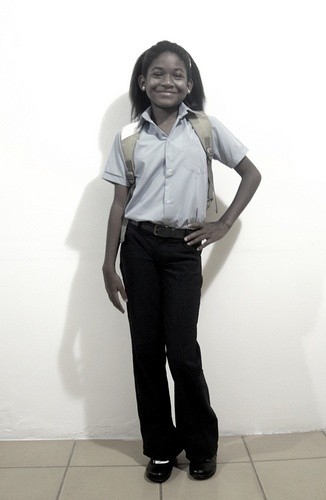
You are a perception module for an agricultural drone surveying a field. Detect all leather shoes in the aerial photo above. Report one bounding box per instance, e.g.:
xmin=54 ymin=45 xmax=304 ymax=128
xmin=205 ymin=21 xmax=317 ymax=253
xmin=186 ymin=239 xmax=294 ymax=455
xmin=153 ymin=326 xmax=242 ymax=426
xmin=146 ymin=457 xmax=174 ymax=483
xmin=189 ymin=454 xmax=218 ymax=480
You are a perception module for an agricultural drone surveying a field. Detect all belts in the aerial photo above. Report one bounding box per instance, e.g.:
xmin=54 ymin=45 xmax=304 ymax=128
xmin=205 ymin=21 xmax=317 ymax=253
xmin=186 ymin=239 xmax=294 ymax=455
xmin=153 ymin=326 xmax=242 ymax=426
xmin=130 ymin=218 xmax=199 ymax=239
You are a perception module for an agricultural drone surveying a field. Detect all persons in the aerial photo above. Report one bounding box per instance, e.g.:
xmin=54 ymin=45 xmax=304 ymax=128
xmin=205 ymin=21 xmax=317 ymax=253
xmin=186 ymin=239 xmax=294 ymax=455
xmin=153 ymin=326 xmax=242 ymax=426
xmin=101 ymin=40 xmax=262 ymax=483
